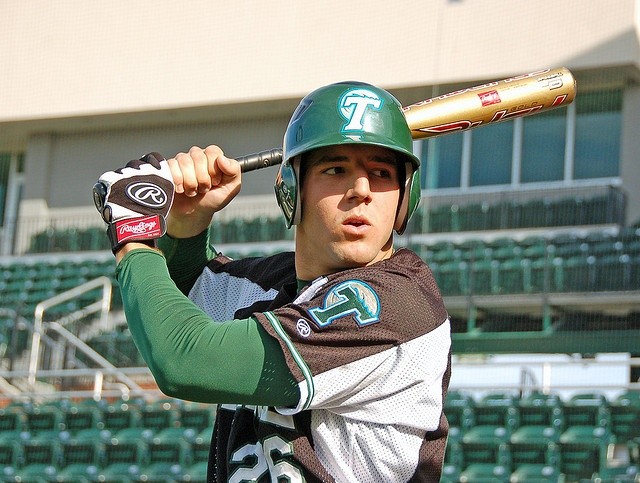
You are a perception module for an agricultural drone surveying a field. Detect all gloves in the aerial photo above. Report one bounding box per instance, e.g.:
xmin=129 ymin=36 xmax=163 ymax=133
xmin=97 ymin=151 xmax=176 ymax=255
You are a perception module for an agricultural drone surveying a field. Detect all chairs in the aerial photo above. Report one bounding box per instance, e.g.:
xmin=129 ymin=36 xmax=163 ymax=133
xmin=224 ymin=250 xmax=286 ymax=261
xmin=23 ymin=226 xmax=112 ymax=253
xmin=0 ymin=257 xmax=124 ymax=358
xmin=393 ymin=226 xmax=640 ymax=297
xmin=407 ymin=195 xmax=623 ymax=233
xmin=76 ymin=323 xmax=149 ymax=368
xmin=0 ymin=395 xmax=216 ymax=483
xmin=209 ymin=215 xmax=295 ymax=245
xmin=441 ymin=388 xmax=640 ymax=483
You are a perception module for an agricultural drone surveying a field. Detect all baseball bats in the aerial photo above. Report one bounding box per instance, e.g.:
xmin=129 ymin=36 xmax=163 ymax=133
xmin=235 ymin=64 xmax=578 ymax=176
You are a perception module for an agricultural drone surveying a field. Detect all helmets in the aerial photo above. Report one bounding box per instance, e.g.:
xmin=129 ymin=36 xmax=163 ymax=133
xmin=273 ymin=80 xmax=421 ymax=235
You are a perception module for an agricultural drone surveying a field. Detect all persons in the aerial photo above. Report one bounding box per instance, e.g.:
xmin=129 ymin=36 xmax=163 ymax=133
xmin=97 ymin=80 xmax=454 ymax=482
